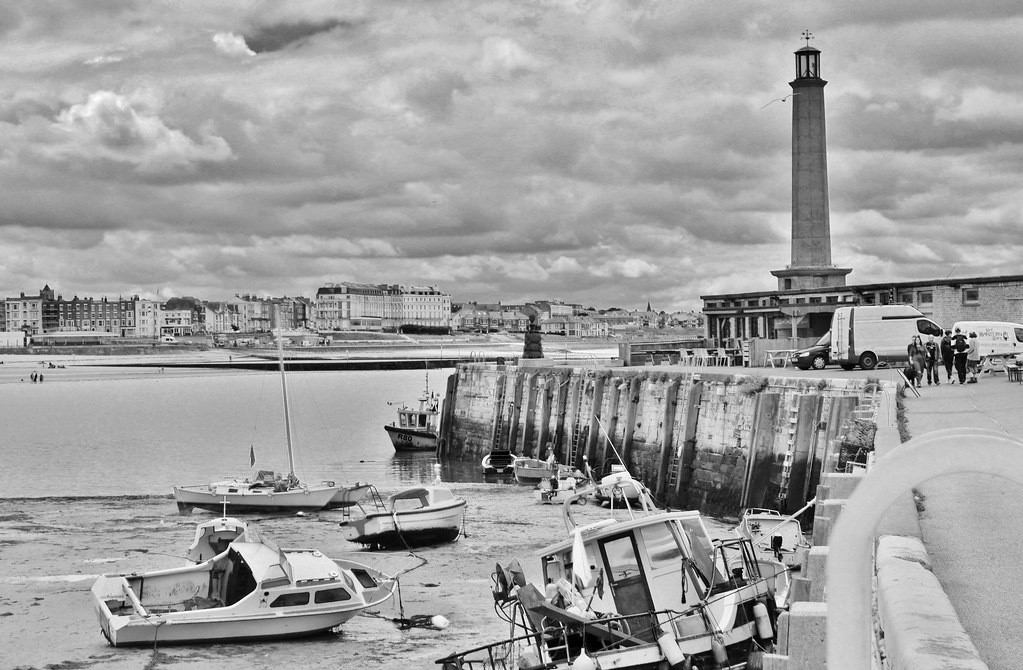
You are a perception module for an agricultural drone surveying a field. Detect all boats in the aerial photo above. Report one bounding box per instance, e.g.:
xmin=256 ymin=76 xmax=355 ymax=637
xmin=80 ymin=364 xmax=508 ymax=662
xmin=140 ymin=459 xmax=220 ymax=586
xmin=588 ymin=455 xmax=648 ymax=507
xmin=534 ymin=465 xmax=601 ymax=506
xmin=482 ymin=448 xmax=518 ymax=475
xmin=90 ymin=520 xmax=399 ymax=647
xmin=513 ymin=454 xmax=576 ymax=485
xmin=435 ymin=415 xmax=810 ymax=670
xmin=339 ymin=486 xmax=467 ymax=553
xmin=382 ymin=373 xmax=441 ymax=452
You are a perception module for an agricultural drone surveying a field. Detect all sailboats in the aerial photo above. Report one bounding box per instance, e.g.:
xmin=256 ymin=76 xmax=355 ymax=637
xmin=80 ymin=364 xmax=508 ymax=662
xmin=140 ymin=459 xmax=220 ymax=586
xmin=172 ymin=325 xmax=374 ymax=511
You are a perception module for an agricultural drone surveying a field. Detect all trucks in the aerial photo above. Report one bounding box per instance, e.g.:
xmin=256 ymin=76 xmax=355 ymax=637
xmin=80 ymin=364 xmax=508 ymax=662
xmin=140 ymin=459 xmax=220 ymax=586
xmin=829 ymin=305 xmax=951 ymax=370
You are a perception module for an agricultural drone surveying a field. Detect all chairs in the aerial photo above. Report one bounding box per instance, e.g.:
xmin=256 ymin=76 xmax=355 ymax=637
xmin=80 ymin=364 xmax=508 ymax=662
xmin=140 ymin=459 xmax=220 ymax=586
xmin=678 ymin=348 xmax=708 ymax=367
xmin=717 ymin=348 xmax=731 ymax=367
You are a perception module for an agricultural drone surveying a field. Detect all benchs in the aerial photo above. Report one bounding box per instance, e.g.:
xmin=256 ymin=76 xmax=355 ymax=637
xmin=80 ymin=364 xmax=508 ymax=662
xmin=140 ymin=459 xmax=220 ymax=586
xmin=762 ymin=356 xmax=796 ymax=360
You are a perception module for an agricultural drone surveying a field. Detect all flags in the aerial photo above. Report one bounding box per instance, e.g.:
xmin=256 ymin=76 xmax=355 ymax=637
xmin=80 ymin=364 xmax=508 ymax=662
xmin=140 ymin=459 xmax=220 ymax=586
xmin=595 ymin=567 xmax=605 ymax=600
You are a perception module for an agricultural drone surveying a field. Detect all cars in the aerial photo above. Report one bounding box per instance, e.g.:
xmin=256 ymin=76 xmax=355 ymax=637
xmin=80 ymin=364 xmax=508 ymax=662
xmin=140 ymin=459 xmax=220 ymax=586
xmin=791 ymin=328 xmax=831 ymax=370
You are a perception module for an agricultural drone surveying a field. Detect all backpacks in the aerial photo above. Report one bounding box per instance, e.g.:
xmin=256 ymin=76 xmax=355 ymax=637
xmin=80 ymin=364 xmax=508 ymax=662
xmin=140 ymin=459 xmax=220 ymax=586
xmin=956 ymin=335 xmax=966 ymax=352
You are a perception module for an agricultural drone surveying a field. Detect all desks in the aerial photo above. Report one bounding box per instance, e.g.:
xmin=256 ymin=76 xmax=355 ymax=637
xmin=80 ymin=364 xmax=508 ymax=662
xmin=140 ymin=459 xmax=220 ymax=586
xmin=762 ymin=349 xmax=799 ymax=369
xmin=713 ymin=348 xmax=741 ymax=366
xmin=688 ymin=356 xmax=715 ymax=367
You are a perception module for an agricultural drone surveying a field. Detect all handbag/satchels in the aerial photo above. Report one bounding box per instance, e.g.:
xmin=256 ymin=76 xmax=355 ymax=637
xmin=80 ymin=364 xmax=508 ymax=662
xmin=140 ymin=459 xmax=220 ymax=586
xmin=906 ymin=364 xmax=917 ymax=379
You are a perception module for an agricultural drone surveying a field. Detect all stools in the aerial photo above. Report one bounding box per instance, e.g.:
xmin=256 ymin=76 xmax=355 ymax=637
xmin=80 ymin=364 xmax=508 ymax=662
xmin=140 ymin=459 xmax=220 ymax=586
xmin=1007 ymin=364 xmax=1018 ymax=382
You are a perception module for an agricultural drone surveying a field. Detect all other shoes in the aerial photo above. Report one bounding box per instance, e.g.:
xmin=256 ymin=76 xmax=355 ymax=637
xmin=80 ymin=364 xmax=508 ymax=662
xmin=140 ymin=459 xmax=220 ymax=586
xmin=917 ymin=384 xmax=921 ymax=387
xmin=951 ymin=380 xmax=955 ymax=384
xmin=947 ymin=381 xmax=951 ymax=383
xmin=960 ymin=379 xmax=965 ymax=384
xmin=967 ymin=379 xmax=977 ymax=383
xmin=937 ymin=382 xmax=940 ymax=385
xmin=929 ymin=382 xmax=931 ymax=385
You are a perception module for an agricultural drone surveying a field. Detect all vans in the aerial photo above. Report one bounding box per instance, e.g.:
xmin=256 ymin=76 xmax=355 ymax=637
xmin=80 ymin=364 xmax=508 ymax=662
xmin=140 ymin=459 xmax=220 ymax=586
xmin=951 ymin=321 xmax=1023 ymax=371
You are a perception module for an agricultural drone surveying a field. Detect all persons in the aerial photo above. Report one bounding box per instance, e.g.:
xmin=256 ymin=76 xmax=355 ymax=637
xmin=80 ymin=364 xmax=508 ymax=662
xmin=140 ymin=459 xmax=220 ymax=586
xmin=907 ymin=335 xmax=925 ymax=388
xmin=924 ymin=334 xmax=941 ymax=386
xmin=966 ymin=331 xmax=980 ymax=384
xmin=951 ymin=328 xmax=970 ymax=384
xmin=940 ymin=330 xmax=955 ymax=384
